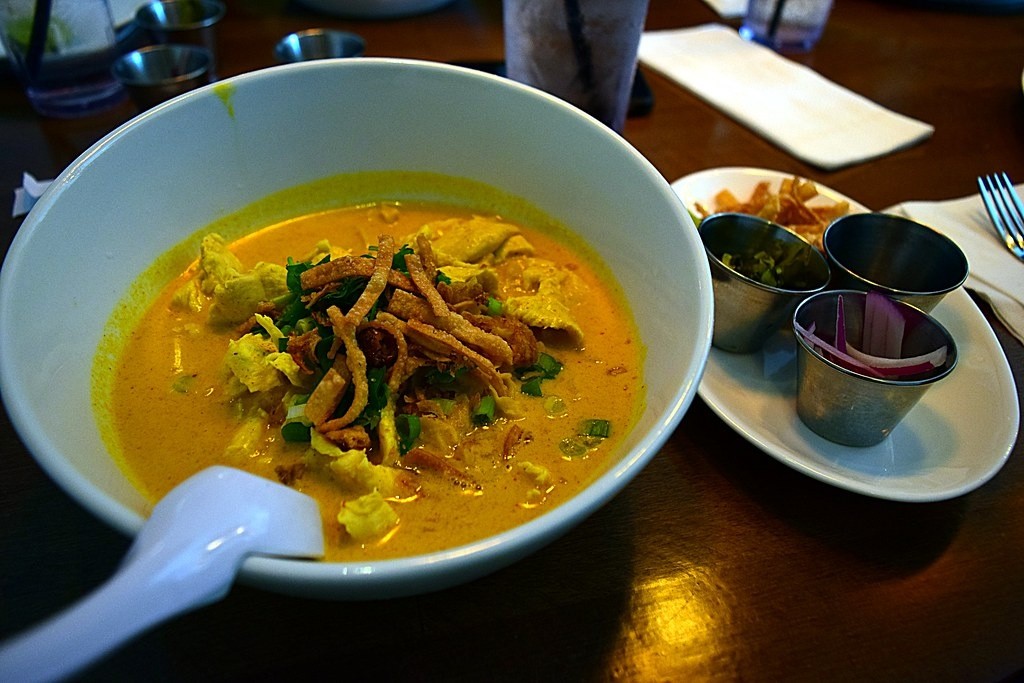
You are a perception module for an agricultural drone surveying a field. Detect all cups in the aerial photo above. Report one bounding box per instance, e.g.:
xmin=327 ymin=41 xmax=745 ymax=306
xmin=135 ymin=1 xmax=227 ymax=74
xmin=502 ymin=0 xmax=649 ymax=137
xmin=112 ymin=45 xmax=216 ymax=114
xmin=740 ymin=0 xmax=832 ymax=54
xmin=0 ymin=0 xmax=125 ymax=118
xmin=275 ymin=28 xmax=366 ymax=64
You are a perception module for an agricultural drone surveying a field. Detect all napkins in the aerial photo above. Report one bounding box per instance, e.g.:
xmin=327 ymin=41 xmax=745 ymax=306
xmin=635 ymin=24 xmax=937 ymax=171
xmin=882 ymin=185 xmax=1024 ymax=344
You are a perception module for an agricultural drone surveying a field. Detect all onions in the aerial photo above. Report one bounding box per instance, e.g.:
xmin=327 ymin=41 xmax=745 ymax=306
xmin=794 ymin=289 xmax=947 ymax=380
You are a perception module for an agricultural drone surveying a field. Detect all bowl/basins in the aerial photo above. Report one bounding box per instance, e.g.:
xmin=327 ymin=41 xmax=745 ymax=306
xmin=697 ymin=211 xmax=830 ymax=354
xmin=823 ymin=213 xmax=968 ymax=314
xmin=792 ymin=289 xmax=958 ymax=448
xmin=0 ymin=57 xmax=714 ymax=600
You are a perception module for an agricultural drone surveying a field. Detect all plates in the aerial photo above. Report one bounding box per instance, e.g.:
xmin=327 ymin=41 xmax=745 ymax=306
xmin=669 ymin=165 xmax=1020 ymax=501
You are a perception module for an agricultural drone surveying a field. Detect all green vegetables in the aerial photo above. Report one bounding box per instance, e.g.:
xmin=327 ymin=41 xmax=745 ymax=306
xmin=247 ymin=245 xmax=454 ymax=430
xmin=678 ymin=212 xmax=804 ymax=291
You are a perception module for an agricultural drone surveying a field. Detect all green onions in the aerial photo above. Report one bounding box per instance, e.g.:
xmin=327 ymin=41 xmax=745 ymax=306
xmin=174 ymin=297 xmax=610 ymax=458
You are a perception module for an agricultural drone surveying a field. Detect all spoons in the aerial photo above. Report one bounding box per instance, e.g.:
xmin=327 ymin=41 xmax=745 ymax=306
xmin=0 ymin=462 xmax=325 ymax=683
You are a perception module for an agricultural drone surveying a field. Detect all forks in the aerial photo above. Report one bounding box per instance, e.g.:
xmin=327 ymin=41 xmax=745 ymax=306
xmin=978 ymin=172 xmax=1024 ymax=264
